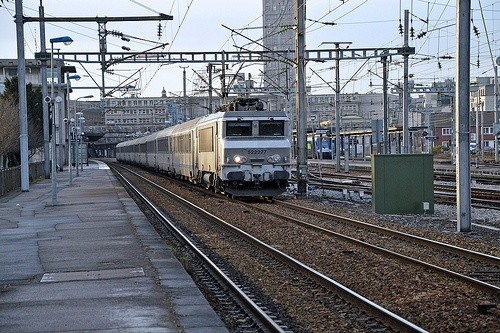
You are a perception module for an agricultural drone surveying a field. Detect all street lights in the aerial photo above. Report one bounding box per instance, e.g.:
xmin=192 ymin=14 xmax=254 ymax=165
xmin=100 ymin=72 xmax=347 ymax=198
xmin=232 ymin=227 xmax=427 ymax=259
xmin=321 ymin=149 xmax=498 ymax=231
xmin=49 ymin=36 xmax=73 ymax=206
xmin=67 ymin=75 xmax=81 ymax=185
xmin=75 ymin=95 xmax=94 ymax=176
xmin=80 ymin=107 xmax=96 ymax=172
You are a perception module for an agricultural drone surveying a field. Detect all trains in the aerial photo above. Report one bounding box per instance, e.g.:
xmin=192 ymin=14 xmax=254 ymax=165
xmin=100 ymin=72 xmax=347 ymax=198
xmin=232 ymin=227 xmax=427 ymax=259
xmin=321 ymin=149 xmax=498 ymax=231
xmin=115 ymin=97 xmax=292 ymax=202
xmin=292 ymin=135 xmax=333 ymax=160
